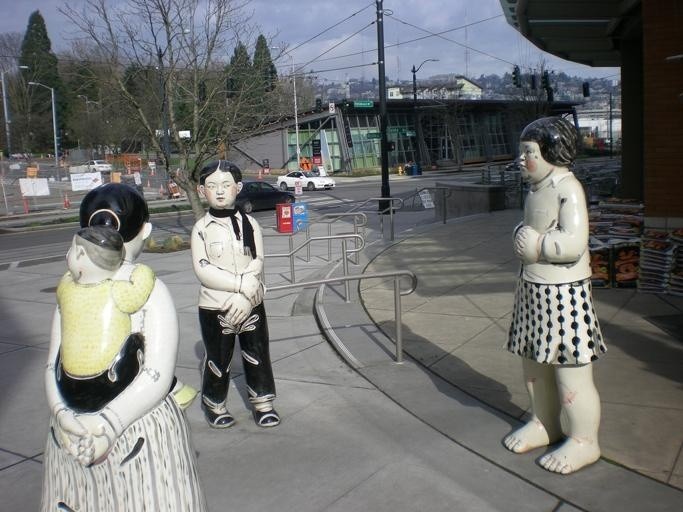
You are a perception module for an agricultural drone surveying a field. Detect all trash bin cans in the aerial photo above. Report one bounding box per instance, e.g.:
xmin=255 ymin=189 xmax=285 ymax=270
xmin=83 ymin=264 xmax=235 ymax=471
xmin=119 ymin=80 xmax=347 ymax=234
xmin=407 ymin=166 xmax=422 ymax=175
xmin=275 ymin=202 xmax=293 ymax=233
xmin=293 ymin=202 xmax=307 ymax=233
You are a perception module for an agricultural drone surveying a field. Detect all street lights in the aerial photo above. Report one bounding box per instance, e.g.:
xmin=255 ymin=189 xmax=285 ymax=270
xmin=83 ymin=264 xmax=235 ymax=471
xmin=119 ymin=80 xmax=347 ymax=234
xmin=77 ymin=95 xmax=90 ymax=123
xmin=157 ymin=29 xmax=191 ymax=172
xmin=29 ymin=81 xmax=60 ymax=183
xmin=411 ymin=58 xmax=440 ymax=176
xmin=1 ymin=65 xmax=29 ymax=161
xmin=63 ymin=189 xmax=71 ymax=209
xmin=271 ymin=46 xmax=300 ymax=170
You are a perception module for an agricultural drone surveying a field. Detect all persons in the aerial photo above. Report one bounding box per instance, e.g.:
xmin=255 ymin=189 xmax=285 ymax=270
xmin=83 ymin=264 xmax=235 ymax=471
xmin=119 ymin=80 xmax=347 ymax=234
xmin=190 ymin=157 xmax=282 ymax=430
xmin=500 ymin=113 xmax=609 ymax=476
xmin=39 ymin=180 xmax=208 ymax=511
xmin=403 ymin=160 xmax=413 ymax=175
xmin=54 ymin=225 xmax=199 ymax=410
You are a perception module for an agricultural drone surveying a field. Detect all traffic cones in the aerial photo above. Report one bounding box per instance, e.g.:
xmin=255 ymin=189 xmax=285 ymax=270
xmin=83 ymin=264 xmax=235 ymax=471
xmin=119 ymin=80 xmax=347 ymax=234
xmin=124 ymin=165 xmax=182 ymax=196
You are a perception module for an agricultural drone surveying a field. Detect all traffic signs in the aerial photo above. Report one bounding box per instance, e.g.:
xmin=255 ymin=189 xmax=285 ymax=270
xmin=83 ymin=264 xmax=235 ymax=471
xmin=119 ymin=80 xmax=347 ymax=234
xmin=388 ymin=128 xmax=409 ymax=133
xmin=353 ymin=100 xmax=373 ymax=108
xmin=368 ymin=133 xmax=382 ymax=139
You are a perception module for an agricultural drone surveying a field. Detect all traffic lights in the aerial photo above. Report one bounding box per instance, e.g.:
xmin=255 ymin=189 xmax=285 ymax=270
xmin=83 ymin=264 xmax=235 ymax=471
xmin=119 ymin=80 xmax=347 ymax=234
xmin=315 ymin=98 xmax=321 ymax=113
xmin=512 ymin=67 xmax=521 ymax=88
xmin=540 ymin=72 xmax=550 ymax=88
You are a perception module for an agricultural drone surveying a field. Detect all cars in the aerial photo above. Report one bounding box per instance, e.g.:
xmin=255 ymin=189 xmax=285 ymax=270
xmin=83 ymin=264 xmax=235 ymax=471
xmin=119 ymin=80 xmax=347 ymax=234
xmin=236 ymin=179 xmax=295 ymax=214
xmin=504 ymin=158 xmax=522 ymax=170
xmin=80 ymin=160 xmax=114 ymax=174
xmin=277 ymin=170 xmax=336 ymax=191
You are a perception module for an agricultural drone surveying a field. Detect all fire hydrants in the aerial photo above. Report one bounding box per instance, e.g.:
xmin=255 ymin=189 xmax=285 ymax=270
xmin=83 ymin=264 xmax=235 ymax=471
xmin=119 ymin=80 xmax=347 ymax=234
xmin=398 ymin=166 xmax=403 ymax=176
